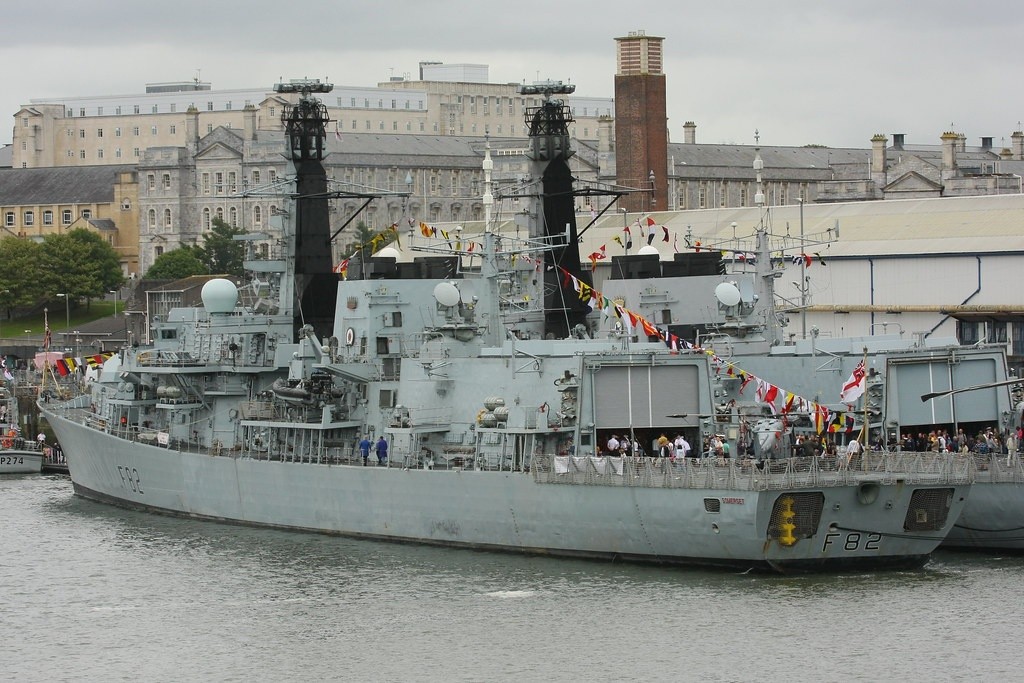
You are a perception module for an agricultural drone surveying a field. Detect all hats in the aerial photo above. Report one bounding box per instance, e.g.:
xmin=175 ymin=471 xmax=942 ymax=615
xmin=891 ymin=432 xmax=896 ymax=435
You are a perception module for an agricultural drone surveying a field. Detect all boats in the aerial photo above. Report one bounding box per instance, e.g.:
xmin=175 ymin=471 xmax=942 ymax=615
xmin=32 ymin=73 xmax=1024 ymax=579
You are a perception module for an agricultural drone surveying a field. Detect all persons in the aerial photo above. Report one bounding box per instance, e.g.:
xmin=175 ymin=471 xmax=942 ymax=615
xmin=359 ymin=436 xmax=371 ymax=465
xmin=879 ymin=426 xmax=1024 ymax=467
xmin=841 ymin=359 xmax=866 ymax=403
xmin=38 ymin=430 xmax=46 ymax=451
xmin=376 ymin=436 xmax=387 ymax=464
xmin=608 ymin=434 xmax=863 ymax=470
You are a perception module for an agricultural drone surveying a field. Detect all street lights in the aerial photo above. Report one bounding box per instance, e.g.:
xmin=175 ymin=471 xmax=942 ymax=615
xmin=110 ymin=290 xmax=116 ymax=318
xmin=57 ymin=294 xmax=70 ymax=349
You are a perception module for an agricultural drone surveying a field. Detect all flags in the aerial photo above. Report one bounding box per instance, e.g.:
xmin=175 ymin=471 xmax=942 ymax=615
xmin=589 ymin=215 xmax=670 ymax=269
xmin=695 ymin=237 xmax=827 ymax=269
xmin=40 ymin=328 xmax=52 ymax=351
xmin=56 ymin=352 xmax=116 ymax=376
xmin=340 ymin=259 xmax=349 ymax=278
xmin=561 ymin=270 xmax=855 ymax=434
xmin=419 ymin=222 xmax=541 ymax=270
